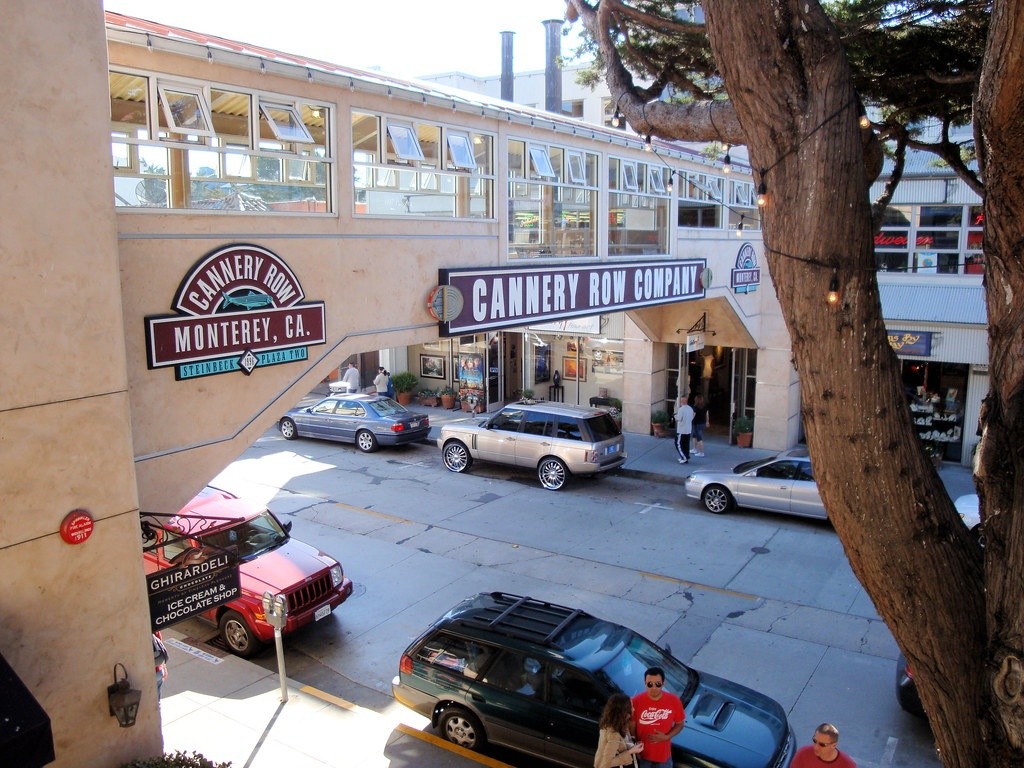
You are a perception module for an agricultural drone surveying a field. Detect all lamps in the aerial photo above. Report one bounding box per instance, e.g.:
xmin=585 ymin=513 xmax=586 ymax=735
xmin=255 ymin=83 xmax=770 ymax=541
xmin=108 ymin=663 xmax=141 ymax=728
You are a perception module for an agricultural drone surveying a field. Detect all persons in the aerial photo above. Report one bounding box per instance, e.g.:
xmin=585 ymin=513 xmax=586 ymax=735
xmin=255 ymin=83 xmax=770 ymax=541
xmin=674 ymin=395 xmax=710 ymax=463
xmin=789 ymin=723 xmax=857 ymax=768
xmin=153 ymin=630 xmax=169 ymax=713
xmin=594 ymin=667 xmax=684 ymax=768
xmin=373 ymin=367 xmax=394 ymax=399
xmin=342 ymin=363 xmax=359 ymax=393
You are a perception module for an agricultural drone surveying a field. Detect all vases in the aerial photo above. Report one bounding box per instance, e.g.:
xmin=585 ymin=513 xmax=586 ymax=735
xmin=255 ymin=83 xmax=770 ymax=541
xmin=419 ymin=398 xmax=440 ymax=407
xmin=553 ymin=370 xmax=560 ymax=385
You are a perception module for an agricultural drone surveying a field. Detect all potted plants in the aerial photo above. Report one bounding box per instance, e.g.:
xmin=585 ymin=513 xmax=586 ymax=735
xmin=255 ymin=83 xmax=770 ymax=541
xmin=460 ymin=395 xmax=486 ymax=414
xmin=391 ymin=372 xmax=418 ymax=405
xmin=651 ymin=411 xmax=669 ymax=436
xmin=735 ymin=417 xmax=753 ymax=448
xmin=441 ymin=385 xmax=457 ymax=409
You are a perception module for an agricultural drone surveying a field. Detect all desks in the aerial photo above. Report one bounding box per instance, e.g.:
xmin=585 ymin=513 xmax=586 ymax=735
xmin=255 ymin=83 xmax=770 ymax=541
xmin=549 ymin=386 xmax=564 ymax=402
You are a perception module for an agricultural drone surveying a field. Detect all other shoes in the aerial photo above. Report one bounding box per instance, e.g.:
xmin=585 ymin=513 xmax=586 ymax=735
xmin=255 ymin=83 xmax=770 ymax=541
xmin=690 ymin=449 xmax=697 ymax=454
xmin=678 ymin=459 xmax=686 ymax=463
xmin=695 ymin=452 xmax=704 ymax=457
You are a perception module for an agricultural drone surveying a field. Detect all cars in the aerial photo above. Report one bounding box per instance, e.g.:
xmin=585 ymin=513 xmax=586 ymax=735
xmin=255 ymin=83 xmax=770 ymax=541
xmin=954 ymin=495 xmax=988 ymax=549
xmin=896 ymin=651 xmax=926 ymax=719
xmin=685 ymin=449 xmax=829 ymax=520
xmin=275 ymin=393 xmax=432 ymax=452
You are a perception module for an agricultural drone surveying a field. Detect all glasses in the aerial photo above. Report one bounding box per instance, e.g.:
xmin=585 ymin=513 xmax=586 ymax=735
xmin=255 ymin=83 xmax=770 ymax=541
xmin=812 ymin=735 xmax=834 ymax=747
xmin=647 ymin=682 xmax=663 ymax=688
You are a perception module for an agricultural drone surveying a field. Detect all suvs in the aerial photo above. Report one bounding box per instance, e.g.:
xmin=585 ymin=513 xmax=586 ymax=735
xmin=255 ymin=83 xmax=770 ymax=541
xmin=142 ymin=485 xmax=352 ymax=658
xmin=392 ymin=592 xmax=797 ymax=768
xmin=436 ymin=400 xmax=628 ymax=491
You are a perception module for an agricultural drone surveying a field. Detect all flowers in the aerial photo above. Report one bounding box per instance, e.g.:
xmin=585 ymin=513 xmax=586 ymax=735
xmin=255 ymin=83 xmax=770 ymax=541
xmin=416 ymin=389 xmax=442 ymax=399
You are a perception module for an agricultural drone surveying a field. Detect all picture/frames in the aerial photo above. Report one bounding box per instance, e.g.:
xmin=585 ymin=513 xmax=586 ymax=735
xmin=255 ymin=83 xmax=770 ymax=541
xmin=561 ymin=356 xmax=587 ymax=382
xmin=534 ymin=343 xmax=551 ymax=384
xmin=419 ymin=354 xmax=446 ymax=380
